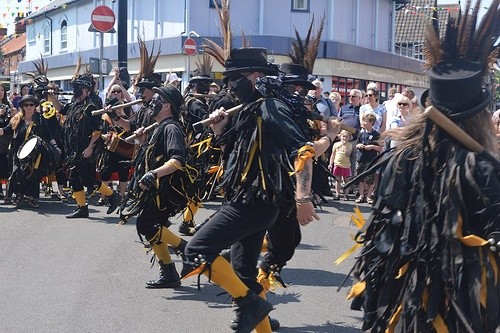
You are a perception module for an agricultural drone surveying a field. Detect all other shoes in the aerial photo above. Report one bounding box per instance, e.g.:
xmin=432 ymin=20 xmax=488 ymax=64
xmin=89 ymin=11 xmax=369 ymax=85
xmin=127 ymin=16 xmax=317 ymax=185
xmin=333 ymin=196 xmax=339 ymax=200
xmin=178 ymin=219 xmax=196 ymax=235
xmin=343 ymin=195 xmax=349 ymax=201
xmin=233 ymin=292 xmax=273 ymax=333
xmin=355 ymin=197 xmax=365 ymax=203
xmin=6 ymin=193 xmax=69 ymax=210
xmin=367 ymin=196 xmax=374 ymax=204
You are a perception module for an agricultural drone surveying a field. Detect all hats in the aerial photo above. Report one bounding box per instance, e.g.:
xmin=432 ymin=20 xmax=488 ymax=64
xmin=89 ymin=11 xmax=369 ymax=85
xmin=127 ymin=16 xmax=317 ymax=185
xmin=33 ymin=47 xmax=316 ymax=116
xmin=17 ymin=95 xmax=39 ymax=107
xmin=420 ymin=61 xmax=492 ymax=118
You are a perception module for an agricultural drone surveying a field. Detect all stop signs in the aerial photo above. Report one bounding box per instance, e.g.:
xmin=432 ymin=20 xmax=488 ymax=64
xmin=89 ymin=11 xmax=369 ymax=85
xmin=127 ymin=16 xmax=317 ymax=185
xmin=90 ymin=5 xmax=117 ymax=32
xmin=183 ymin=37 xmax=197 ymax=56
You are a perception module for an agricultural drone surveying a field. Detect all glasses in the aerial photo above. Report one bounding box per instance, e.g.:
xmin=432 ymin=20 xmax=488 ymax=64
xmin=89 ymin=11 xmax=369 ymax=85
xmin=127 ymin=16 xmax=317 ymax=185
xmin=111 ymin=91 xmax=122 ymax=94
xmin=398 ymin=103 xmax=409 ymax=106
xmin=23 ymin=103 xmax=35 ymax=107
xmin=366 ymin=95 xmax=375 ymax=97
xmin=348 ymin=95 xmax=358 ymax=98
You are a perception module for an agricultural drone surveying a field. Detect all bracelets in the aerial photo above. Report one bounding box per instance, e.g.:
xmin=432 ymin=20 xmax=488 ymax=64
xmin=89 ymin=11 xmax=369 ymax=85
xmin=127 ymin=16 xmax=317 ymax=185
xmin=294 ymin=196 xmax=312 ymax=205
xmin=324 ymin=133 xmax=332 ymax=146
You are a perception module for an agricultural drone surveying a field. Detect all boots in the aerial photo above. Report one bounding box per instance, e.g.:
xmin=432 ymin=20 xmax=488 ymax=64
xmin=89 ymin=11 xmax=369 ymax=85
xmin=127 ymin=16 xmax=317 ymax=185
xmin=105 ymin=191 xmax=122 ymax=214
xmin=173 ymin=239 xmax=198 ymax=276
xmin=65 ymin=204 xmax=88 ymax=218
xmin=146 ymin=260 xmax=181 ymax=287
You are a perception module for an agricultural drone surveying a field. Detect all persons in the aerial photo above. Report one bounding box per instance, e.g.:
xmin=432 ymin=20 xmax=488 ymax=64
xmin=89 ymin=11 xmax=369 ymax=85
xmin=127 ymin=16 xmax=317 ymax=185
xmin=334 ymin=55 xmax=500 ymax=333
xmin=133 ymin=84 xmax=206 ymax=288
xmin=0 ymin=64 xmax=433 ymax=236
xmin=185 ymin=47 xmax=320 ymax=333
xmin=218 ymin=64 xmax=344 ymax=295
xmin=487 ymin=108 xmax=500 ymax=162
